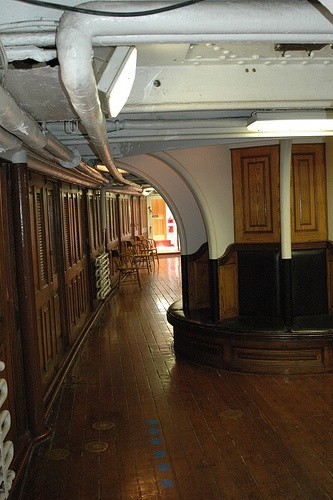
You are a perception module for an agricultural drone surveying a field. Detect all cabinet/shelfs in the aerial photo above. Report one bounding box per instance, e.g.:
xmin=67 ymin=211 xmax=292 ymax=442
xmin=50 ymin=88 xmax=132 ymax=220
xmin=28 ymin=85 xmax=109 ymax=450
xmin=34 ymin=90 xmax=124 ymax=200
xmin=15 ymin=167 xmax=161 ymax=436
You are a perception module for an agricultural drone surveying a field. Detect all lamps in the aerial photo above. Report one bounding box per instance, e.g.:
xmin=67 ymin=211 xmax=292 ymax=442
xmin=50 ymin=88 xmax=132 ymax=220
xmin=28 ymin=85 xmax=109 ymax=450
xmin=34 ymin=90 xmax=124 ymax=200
xmin=93 ymin=44 xmax=137 ymax=119
xmin=245 ymin=109 xmax=333 ymax=137
